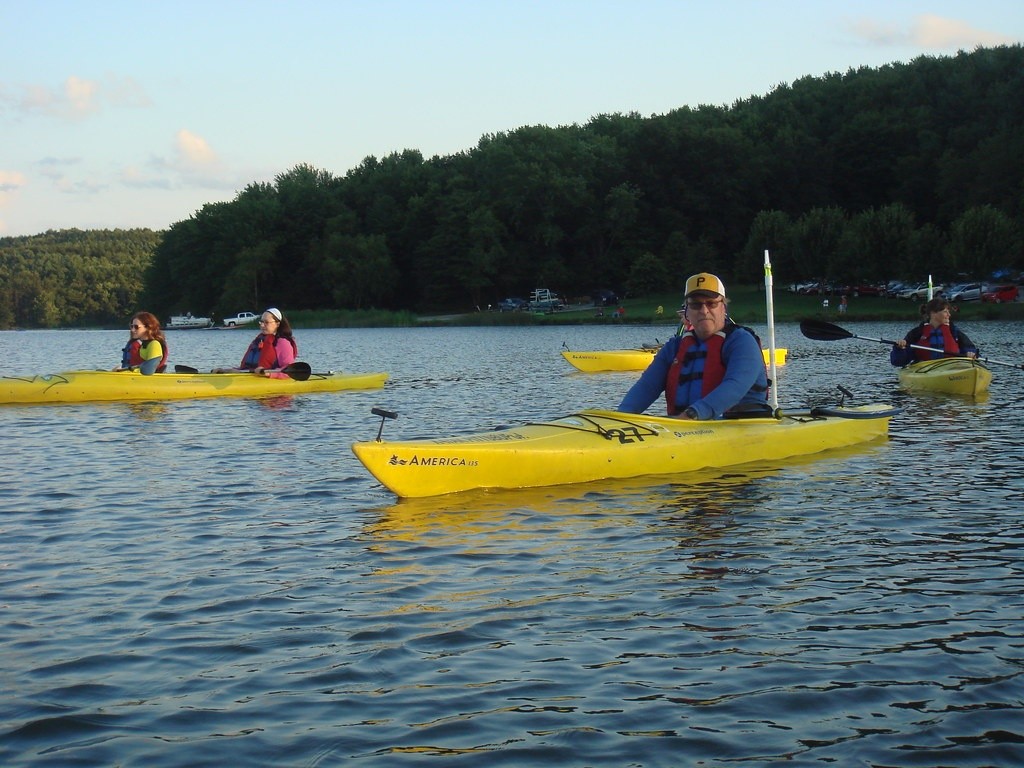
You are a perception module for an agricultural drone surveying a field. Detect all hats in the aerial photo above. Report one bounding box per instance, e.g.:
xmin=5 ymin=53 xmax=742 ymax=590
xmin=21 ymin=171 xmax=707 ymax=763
xmin=684 ymin=272 xmax=726 ymax=299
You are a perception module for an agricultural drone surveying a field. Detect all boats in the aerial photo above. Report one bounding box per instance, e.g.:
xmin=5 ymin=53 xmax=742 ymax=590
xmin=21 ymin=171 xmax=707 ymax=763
xmin=560 ymin=340 xmax=790 ymax=373
xmin=899 ymin=356 xmax=992 ymax=397
xmin=350 ymin=384 xmax=905 ymax=499
xmin=161 ymin=312 xmax=215 ymax=331
xmin=1 ymin=368 xmax=386 ymax=403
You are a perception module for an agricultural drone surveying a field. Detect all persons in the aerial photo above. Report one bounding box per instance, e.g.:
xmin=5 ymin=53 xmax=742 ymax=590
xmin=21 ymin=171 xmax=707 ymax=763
xmin=616 ymin=273 xmax=774 ymax=419
xmin=952 ymin=304 xmax=960 ymax=313
xmin=121 ymin=311 xmax=169 ymax=377
xmin=889 ymin=298 xmax=982 ymax=368
xmin=841 ymin=295 xmax=847 ymax=313
xmin=212 ymin=306 xmax=298 ymax=380
xmin=612 ymin=306 xmax=624 ymax=317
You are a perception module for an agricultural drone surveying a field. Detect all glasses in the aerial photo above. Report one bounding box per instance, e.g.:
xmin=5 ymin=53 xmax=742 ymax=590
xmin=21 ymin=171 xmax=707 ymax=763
xmin=130 ymin=324 xmax=147 ymax=330
xmin=258 ymin=320 xmax=277 ymax=325
xmin=687 ymin=300 xmax=722 ymax=310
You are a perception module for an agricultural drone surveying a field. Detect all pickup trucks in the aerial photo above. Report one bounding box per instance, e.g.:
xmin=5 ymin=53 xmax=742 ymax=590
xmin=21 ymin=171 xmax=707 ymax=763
xmin=223 ymin=311 xmax=262 ymax=327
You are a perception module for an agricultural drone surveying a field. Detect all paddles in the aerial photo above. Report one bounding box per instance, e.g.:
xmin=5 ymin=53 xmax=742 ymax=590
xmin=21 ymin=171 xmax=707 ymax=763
xmin=800 ymin=318 xmax=1023 ymax=374
xmin=174 ymin=361 xmax=312 ymax=381
xmin=730 ymin=402 xmax=908 ymax=421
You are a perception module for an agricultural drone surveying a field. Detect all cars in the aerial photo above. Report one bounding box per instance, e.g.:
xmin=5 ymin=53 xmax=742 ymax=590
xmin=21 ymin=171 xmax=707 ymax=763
xmin=940 ymin=283 xmax=989 ymax=303
xmin=787 ymin=270 xmax=948 ymax=304
xmin=982 ymin=284 xmax=1020 ymax=304
